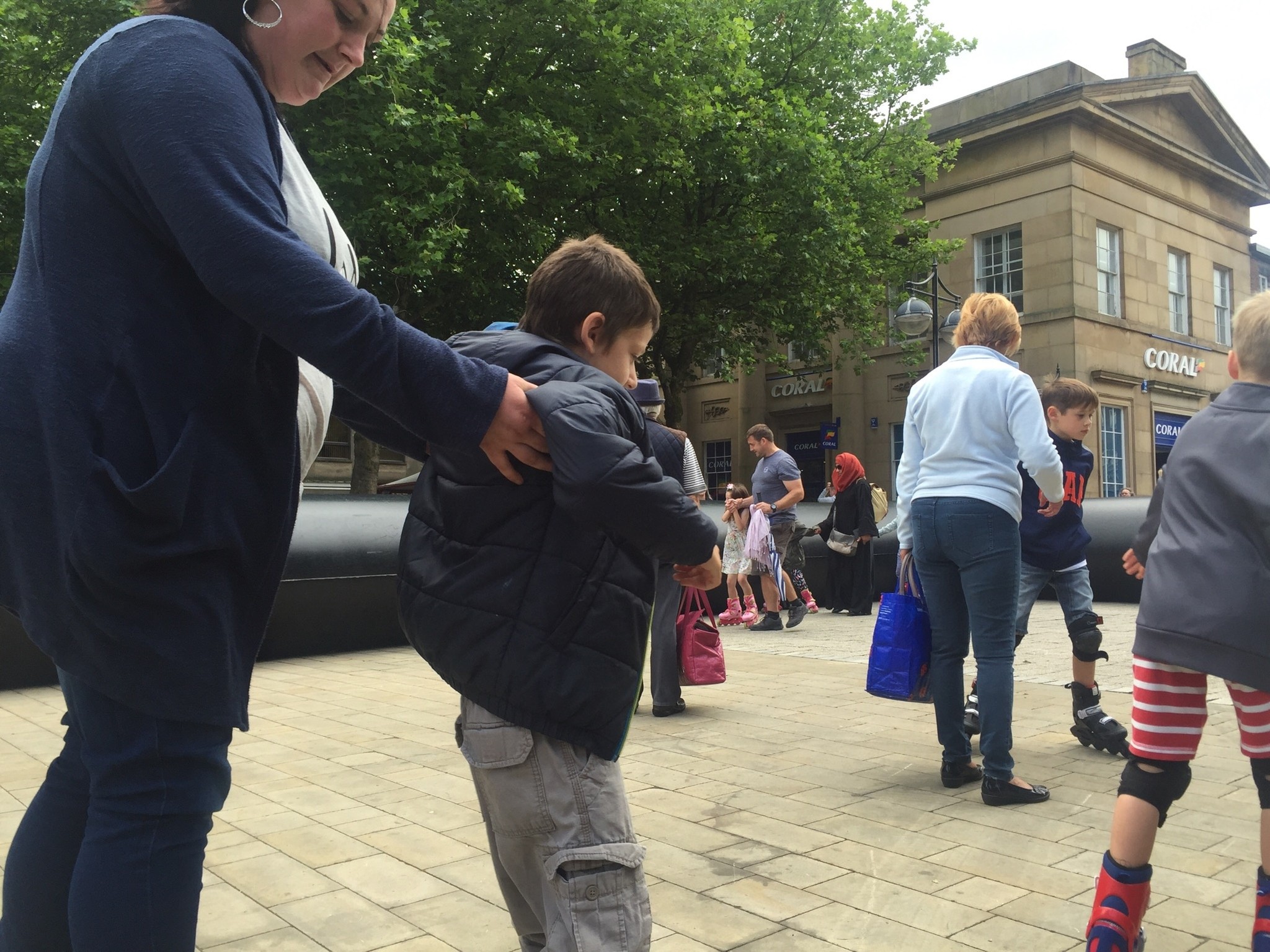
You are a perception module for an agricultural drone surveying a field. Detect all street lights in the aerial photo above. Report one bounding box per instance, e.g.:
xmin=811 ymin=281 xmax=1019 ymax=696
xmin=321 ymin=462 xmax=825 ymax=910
xmin=893 ymin=252 xmax=965 ymax=370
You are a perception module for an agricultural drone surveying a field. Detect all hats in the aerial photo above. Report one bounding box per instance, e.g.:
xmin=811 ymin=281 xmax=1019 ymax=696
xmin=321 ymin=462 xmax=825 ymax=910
xmin=627 ymin=378 xmax=665 ymax=406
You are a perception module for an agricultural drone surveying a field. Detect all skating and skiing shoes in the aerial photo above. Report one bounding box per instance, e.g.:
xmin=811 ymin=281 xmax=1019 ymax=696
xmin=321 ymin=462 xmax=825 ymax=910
xmin=741 ymin=593 xmax=759 ymax=629
xmin=761 ymin=597 xmax=782 ymax=613
xmin=963 ymin=678 xmax=981 ymax=740
xmin=1250 ymin=866 xmax=1270 ymax=952
xmin=801 ymin=589 xmax=819 ymax=613
xmin=1086 ymin=849 xmax=1153 ymax=952
xmin=716 ymin=598 xmax=742 ymax=626
xmin=1065 ymin=681 xmax=1134 ymax=758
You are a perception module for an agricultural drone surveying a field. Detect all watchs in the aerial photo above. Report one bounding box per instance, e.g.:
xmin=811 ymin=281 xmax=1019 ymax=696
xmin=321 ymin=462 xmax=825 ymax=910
xmin=770 ymin=503 xmax=777 ymax=513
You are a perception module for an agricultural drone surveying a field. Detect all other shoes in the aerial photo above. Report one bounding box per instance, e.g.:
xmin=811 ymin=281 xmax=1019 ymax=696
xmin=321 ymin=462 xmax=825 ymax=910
xmin=940 ymin=760 xmax=983 ymax=788
xmin=786 ymin=599 xmax=808 ymax=629
xmin=832 ymin=607 xmax=843 ymax=613
xmin=652 ymin=697 xmax=686 ymax=716
xmin=847 ymin=610 xmax=866 ymax=615
xmin=981 ymin=777 xmax=1050 ymax=806
xmin=749 ymin=610 xmax=784 ymax=631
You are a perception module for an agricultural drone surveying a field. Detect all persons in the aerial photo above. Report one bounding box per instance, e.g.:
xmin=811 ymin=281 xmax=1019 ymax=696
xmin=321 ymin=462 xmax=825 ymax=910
xmin=720 ymin=424 xmax=902 ymax=633
xmin=896 ymin=294 xmax=1065 ymax=807
xmin=1078 ymin=292 xmax=1270 ymax=952
xmin=0 ymin=0 xmax=554 ymax=952
xmin=397 ymin=231 xmax=723 ymax=952
xmin=962 ymin=374 xmax=1128 ymax=742
xmin=627 ymin=377 xmax=707 ymax=718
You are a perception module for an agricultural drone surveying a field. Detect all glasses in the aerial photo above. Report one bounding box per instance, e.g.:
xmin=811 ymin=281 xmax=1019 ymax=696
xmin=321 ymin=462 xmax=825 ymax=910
xmin=835 ymin=465 xmax=842 ymax=470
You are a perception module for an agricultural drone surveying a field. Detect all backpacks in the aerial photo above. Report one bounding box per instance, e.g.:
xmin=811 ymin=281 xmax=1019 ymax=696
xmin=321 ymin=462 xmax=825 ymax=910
xmin=856 ymin=477 xmax=888 ymax=524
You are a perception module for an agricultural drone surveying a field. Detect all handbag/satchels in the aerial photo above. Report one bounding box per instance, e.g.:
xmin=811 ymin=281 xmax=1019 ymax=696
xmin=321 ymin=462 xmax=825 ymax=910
xmin=864 ymin=552 xmax=934 ymax=704
xmin=827 ymin=528 xmax=858 ymax=557
xmin=675 ymin=584 xmax=726 ymax=687
